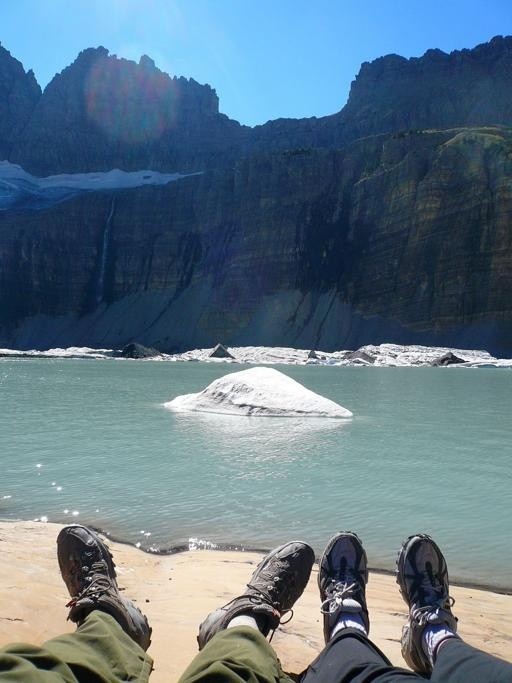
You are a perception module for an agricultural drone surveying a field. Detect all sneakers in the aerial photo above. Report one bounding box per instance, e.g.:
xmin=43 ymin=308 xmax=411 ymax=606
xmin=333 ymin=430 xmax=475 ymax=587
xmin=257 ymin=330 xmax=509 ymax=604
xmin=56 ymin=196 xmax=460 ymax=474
xmin=197 ymin=541 xmax=315 ymax=651
xmin=317 ymin=529 xmax=370 ymax=645
xmin=56 ymin=525 xmax=153 ymax=652
xmin=395 ymin=534 xmax=457 ymax=673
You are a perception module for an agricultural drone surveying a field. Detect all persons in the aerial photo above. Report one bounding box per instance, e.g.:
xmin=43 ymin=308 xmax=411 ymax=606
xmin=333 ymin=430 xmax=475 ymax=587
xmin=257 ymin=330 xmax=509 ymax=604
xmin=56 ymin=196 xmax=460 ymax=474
xmin=1 ymin=525 xmax=316 ymax=683
xmin=297 ymin=530 xmax=512 ymax=683
